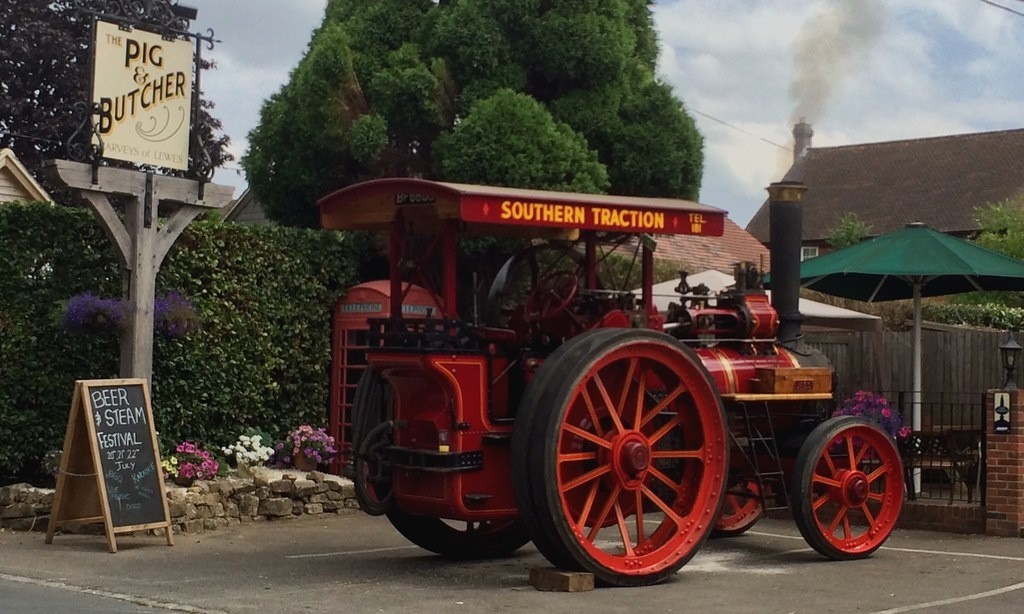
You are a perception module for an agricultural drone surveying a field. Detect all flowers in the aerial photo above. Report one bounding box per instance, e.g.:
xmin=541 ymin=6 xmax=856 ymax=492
xmin=832 ymin=390 xmax=913 ymax=437
xmin=55 ymin=293 xmax=137 ymax=342
xmin=176 ymin=442 xmax=230 ymax=485
xmin=221 ymin=434 xmax=276 ymax=468
xmin=270 ymin=424 xmax=337 ymax=471
xmin=156 ymin=430 xmax=179 ymax=482
xmin=154 ymin=290 xmax=199 ymax=341
xmin=42 ymin=450 xmax=63 ymax=480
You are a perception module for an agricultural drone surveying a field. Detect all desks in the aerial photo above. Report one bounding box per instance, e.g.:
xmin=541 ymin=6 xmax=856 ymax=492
xmin=861 ymin=459 xmax=969 ymax=495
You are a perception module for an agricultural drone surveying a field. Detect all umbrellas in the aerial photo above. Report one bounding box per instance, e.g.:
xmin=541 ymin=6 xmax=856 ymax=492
xmin=760 ymin=223 xmax=1023 ymax=498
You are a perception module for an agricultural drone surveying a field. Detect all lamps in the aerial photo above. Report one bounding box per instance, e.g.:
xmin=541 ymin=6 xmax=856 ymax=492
xmin=998 ymin=331 xmax=1022 ymax=390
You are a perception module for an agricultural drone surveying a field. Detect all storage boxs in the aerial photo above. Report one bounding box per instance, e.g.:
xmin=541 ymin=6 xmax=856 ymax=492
xmin=753 ymin=367 xmax=831 ymax=393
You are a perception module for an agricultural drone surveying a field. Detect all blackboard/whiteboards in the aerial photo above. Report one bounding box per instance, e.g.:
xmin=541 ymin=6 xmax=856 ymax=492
xmin=43 ymin=375 xmax=175 ymax=555
